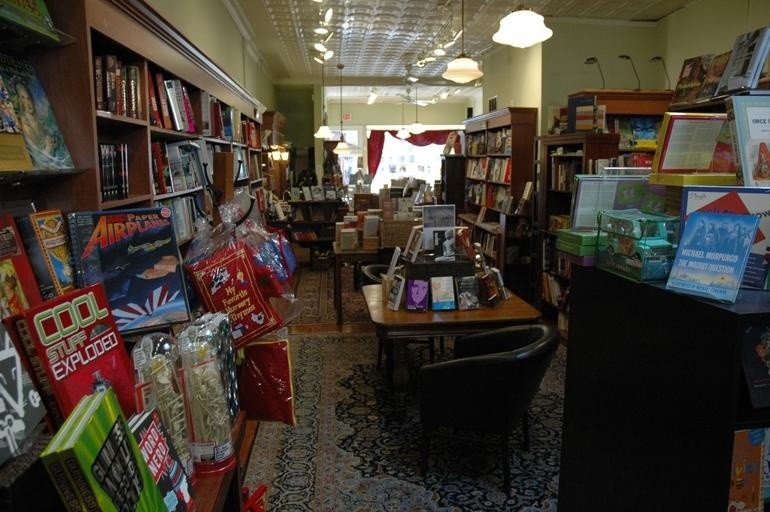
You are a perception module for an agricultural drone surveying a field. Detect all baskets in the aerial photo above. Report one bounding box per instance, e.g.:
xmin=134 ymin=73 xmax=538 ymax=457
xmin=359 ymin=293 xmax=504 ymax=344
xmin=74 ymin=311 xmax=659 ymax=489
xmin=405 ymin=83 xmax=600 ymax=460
xmin=378 ymin=217 xmax=422 ymax=249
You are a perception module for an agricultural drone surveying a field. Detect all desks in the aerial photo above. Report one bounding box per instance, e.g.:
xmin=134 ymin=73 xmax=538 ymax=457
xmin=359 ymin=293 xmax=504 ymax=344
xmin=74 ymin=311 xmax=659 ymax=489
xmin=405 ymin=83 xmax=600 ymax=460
xmin=645 ymin=278 xmax=769 ymax=353
xmin=361 ymin=283 xmax=543 ymax=367
xmin=331 ymin=242 xmax=410 ymax=329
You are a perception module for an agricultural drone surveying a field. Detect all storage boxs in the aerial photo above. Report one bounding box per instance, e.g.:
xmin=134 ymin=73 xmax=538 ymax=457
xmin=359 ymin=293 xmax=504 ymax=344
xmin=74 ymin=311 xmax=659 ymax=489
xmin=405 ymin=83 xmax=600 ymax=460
xmin=554 ymin=228 xmax=608 ymax=268
xmin=595 ymin=207 xmax=682 ymax=283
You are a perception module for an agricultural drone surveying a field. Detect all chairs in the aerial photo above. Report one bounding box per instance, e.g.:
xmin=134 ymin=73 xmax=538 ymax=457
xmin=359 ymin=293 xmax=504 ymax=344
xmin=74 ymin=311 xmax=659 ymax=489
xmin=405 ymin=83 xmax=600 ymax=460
xmin=417 ymin=324 xmax=552 ymax=496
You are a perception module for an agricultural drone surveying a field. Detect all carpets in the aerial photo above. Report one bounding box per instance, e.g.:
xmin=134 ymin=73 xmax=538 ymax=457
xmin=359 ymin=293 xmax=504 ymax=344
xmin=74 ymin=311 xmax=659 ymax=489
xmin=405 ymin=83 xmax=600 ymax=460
xmin=240 ymin=330 xmax=567 ymax=512
xmin=288 ymin=259 xmax=375 ymax=326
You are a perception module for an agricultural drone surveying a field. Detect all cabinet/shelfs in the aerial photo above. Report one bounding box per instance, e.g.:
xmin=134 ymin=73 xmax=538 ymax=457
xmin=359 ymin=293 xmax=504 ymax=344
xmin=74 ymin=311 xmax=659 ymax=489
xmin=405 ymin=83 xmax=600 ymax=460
xmin=453 ymin=105 xmax=539 ymax=284
xmin=566 ymin=89 xmax=679 ymax=167
xmin=0 ymin=0 xmax=289 ymax=270
xmin=538 ymin=132 xmax=623 ymax=313
